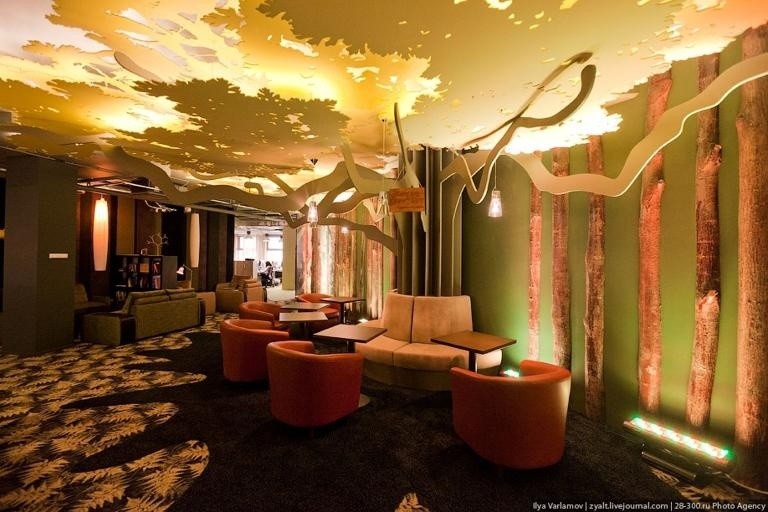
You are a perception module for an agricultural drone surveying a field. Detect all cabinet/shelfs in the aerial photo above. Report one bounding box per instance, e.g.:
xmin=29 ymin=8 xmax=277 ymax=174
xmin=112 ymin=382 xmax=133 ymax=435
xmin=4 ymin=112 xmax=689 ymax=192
xmin=113 ymin=255 xmax=163 ymax=300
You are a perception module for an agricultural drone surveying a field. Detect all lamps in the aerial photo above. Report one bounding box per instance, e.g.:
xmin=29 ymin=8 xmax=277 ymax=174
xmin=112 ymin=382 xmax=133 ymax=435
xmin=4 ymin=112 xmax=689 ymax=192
xmin=307 ymin=203 xmax=320 ymax=226
xmin=93 ymin=194 xmax=109 ymax=272
xmin=622 ymin=417 xmax=739 ymax=473
xmin=486 ymin=158 xmax=504 ymax=217
xmin=190 ymin=210 xmax=201 ymax=269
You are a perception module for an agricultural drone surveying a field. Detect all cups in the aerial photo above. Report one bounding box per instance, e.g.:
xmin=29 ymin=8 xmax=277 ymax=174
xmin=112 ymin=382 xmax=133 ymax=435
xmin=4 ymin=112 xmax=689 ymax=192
xmin=141 ymin=248 xmax=147 ymax=255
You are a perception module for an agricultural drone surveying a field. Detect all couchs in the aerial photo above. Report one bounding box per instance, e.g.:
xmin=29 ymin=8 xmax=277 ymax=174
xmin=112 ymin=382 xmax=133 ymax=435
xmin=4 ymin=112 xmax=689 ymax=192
xmin=76 ymin=288 xmax=207 ymax=344
xmin=355 ymin=287 xmax=503 ymax=391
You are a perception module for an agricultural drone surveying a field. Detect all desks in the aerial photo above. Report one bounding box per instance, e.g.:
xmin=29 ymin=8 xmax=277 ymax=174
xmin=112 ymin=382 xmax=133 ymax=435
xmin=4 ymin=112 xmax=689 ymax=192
xmin=430 ymin=330 xmax=516 ymax=372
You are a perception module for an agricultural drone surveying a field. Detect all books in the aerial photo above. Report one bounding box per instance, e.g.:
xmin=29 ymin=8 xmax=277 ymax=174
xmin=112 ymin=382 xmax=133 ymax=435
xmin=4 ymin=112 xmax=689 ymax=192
xmin=114 ymin=254 xmax=162 ymax=304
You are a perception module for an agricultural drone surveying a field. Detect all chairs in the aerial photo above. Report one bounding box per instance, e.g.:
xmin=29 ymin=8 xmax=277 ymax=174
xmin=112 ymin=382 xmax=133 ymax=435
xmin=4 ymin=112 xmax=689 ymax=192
xmin=450 ymin=360 xmax=572 ymax=469
xmin=216 ymin=275 xmax=388 ymax=428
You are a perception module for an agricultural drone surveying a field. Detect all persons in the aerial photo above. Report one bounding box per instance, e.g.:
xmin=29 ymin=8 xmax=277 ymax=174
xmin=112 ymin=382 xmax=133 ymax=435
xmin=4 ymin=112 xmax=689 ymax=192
xmin=257 ymin=261 xmax=273 ymax=276
